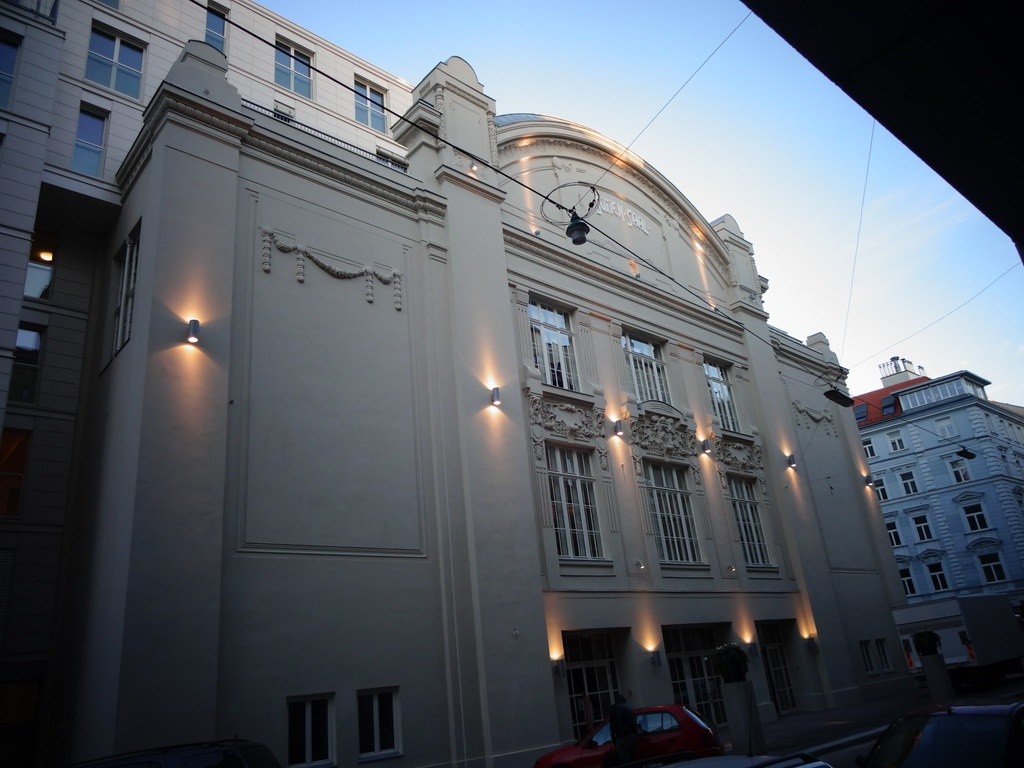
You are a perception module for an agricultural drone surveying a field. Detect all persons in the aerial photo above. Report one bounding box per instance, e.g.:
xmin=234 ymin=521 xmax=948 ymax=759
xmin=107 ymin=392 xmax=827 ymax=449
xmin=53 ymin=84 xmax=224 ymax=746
xmin=609 ymin=695 xmax=638 ymax=763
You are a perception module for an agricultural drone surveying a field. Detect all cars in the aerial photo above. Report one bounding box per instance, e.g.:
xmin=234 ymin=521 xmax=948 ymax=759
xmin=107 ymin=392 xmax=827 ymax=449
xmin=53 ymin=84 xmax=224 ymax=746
xmin=62 ymin=734 xmax=284 ymax=768
xmin=853 ymin=701 xmax=1024 ymax=768
xmin=644 ymin=754 xmax=835 ymax=768
xmin=531 ymin=704 xmax=727 ymax=768
